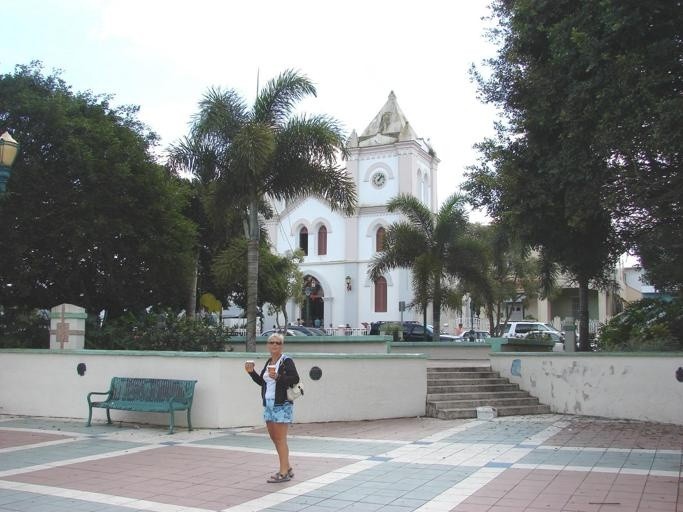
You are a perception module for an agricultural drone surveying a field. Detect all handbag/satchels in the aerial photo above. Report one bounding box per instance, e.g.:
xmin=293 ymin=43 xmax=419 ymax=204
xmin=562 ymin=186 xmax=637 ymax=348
xmin=287 ymin=382 xmax=305 ymax=402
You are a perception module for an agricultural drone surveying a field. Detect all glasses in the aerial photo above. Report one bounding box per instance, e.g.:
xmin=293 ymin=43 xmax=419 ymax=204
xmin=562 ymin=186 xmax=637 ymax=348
xmin=268 ymin=341 xmax=283 ymax=345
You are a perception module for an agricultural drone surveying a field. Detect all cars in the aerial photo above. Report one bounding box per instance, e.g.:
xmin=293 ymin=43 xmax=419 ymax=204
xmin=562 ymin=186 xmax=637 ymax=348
xmin=457 ymin=329 xmax=489 ymax=342
xmin=520 ymin=329 xmax=579 ymax=352
xmin=260 ymin=323 xmax=333 ymax=336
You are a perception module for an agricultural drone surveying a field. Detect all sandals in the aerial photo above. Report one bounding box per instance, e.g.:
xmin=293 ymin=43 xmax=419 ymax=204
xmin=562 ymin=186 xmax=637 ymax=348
xmin=266 ymin=468 xmax=293 ymax=484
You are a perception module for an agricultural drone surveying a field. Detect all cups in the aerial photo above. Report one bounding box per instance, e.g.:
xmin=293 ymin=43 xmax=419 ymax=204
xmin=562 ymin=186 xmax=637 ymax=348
xmin=267 ymin=366 xmax=275 ymax=377
xmin=245 ymin=361 xmax=253 ymax=372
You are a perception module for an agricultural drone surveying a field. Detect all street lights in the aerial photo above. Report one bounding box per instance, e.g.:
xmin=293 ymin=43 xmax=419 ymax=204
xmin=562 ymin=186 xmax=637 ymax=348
xmin=303 ymin=286 xmax=312 ymax=328
xmin=0 ymin=129 xmax=21 ymax=196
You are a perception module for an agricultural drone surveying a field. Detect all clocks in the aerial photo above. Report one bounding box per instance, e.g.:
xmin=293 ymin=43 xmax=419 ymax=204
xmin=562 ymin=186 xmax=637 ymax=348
xmin=370 ymin=170 xmax=387 ymax=190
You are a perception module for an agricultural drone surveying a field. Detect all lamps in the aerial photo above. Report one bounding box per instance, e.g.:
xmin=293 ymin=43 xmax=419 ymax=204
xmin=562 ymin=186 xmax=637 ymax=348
xmin=345 ymin=276 xmax=351 ymax=291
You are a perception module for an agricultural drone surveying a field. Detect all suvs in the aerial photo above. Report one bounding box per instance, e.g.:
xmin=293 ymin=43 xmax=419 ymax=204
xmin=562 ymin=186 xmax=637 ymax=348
xmin=367 ymin=319 xmax=463 ymax=342
xmin=492 ymin=320 xmax=579 ymax=344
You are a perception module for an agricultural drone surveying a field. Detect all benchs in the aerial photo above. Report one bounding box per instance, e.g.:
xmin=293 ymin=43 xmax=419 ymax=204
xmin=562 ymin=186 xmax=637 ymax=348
xmin=87 ymin=377 xmax=198 ymax=434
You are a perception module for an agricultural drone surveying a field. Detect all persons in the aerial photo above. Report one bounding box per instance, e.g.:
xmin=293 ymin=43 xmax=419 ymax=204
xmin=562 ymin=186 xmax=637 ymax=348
xmin=296 ymin=317 xmax=304 ymax=326
xmin=271 ymin=324 xmax=277 ymax=331
xmin=343 ymin=323 xmax=351 ymax=335
xmin=327 ymin=324 xmax=334 ymax=335
xmin=243 ymin=332 xmax=300 ymax=483
xmin=558 ymin=331 xmax=565 ymax=344
xmin=455 ymin=323 xmax=464 ymax=336
xmin=314 ymin=318 xmax=321 ymax=329
